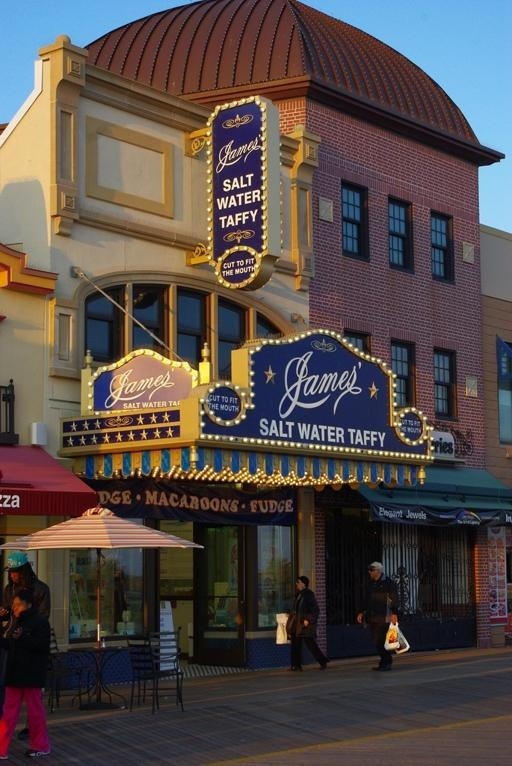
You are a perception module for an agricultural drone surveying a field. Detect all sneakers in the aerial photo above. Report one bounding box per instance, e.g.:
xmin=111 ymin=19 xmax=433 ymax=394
xmin=26 ymin=748 xmax=50 ymax=756
xmin=372 ymin=662 xmax=392 ymax=670
xmin=285 ymin=665 xmax=302 ymax=671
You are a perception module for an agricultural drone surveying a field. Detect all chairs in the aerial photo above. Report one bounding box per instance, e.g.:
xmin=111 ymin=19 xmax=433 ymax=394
xmin=44 ymin=628 xmax=89 ymax=711
xmin=127 ymin=629 xmax=185 ymax=716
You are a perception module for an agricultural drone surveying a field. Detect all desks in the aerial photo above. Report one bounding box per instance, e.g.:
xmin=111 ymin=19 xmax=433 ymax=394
xmin=68 ymin=646 xmax=127 ymax=708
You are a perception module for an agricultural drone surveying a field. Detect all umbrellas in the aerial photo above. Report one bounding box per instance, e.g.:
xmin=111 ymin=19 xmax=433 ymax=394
xmin=0 ymin=505 xmax=207 ymax=647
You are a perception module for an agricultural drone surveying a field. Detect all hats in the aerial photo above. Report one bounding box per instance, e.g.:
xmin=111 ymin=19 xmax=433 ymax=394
xmin=7 ymin=550 xmax=28 ymax=569
xmin=297 ymin=575 xmax=310 ymax=587
xmin=368 ymin=561 xmax=385 ymax=574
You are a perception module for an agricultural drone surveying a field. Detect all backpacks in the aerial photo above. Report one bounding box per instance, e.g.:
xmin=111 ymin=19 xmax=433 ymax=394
xmin=384 ymin=621 xmax=411 ymax=655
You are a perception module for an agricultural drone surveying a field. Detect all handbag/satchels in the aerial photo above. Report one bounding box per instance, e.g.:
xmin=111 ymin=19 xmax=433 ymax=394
xmin=274 ymin=613 xmax=296 ymax=644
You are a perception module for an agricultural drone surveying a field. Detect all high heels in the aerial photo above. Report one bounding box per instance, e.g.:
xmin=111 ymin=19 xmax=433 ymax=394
xmin=320 ymin=657 xmax=330 ymax=670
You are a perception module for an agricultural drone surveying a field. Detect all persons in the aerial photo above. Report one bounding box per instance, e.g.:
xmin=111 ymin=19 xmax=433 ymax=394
xmin=0 ymin=551 xmax=54 ymax=759
xmin=356 ymin=561 xmax=402 ymax=671
xmin=287 ymin=575 xmax=328 ymax=672
